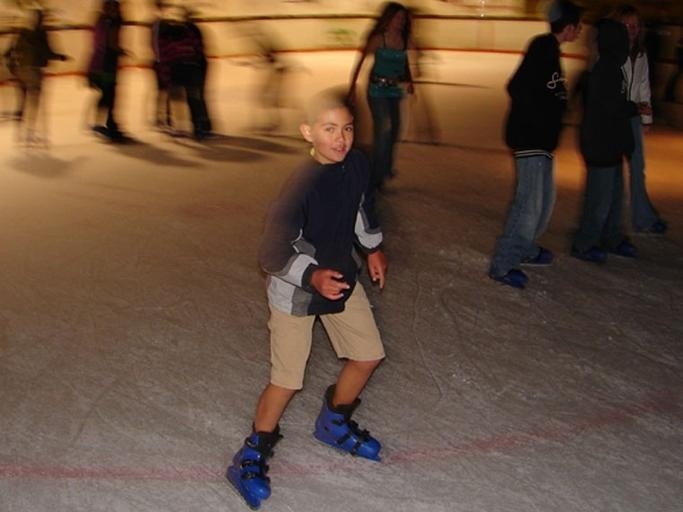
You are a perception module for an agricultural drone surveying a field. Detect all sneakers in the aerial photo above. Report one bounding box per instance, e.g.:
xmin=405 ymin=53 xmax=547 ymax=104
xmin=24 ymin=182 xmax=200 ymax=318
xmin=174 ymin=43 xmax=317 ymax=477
xmin=632 ymin=216 xmax=667 ymax=234
xmin=568 ymin=241 xmax=606 ymax=264
xmin=487 ymin=266 xmax=528 ymax=286
xmin=611 ymin=236 xmax=636 ymax=256
xmin=522 ymin=243 xmax=554 ymax=265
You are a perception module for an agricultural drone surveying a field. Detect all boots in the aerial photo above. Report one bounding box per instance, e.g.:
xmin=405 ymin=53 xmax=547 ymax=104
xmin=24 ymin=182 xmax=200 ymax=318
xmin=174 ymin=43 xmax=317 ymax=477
xmin=230 ymin=421 xmax=284 ymax=501
xmin=316 ymin=385 xmax=381 ymax=457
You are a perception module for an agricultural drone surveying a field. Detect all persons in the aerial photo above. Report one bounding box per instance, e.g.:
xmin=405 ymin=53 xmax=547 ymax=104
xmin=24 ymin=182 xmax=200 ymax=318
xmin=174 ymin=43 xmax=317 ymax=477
xmin=487 ymin=0 xmax=583 ymax=289
xmin=223 ymin=88 xmax=387 ymax=508
xmin=0 ymin=0 xmax=213 ymax=149
xmin=344 ymin=2 xmax=416 ymax=192
xmin=567 ymin=1 xmax=682 ymax=263
